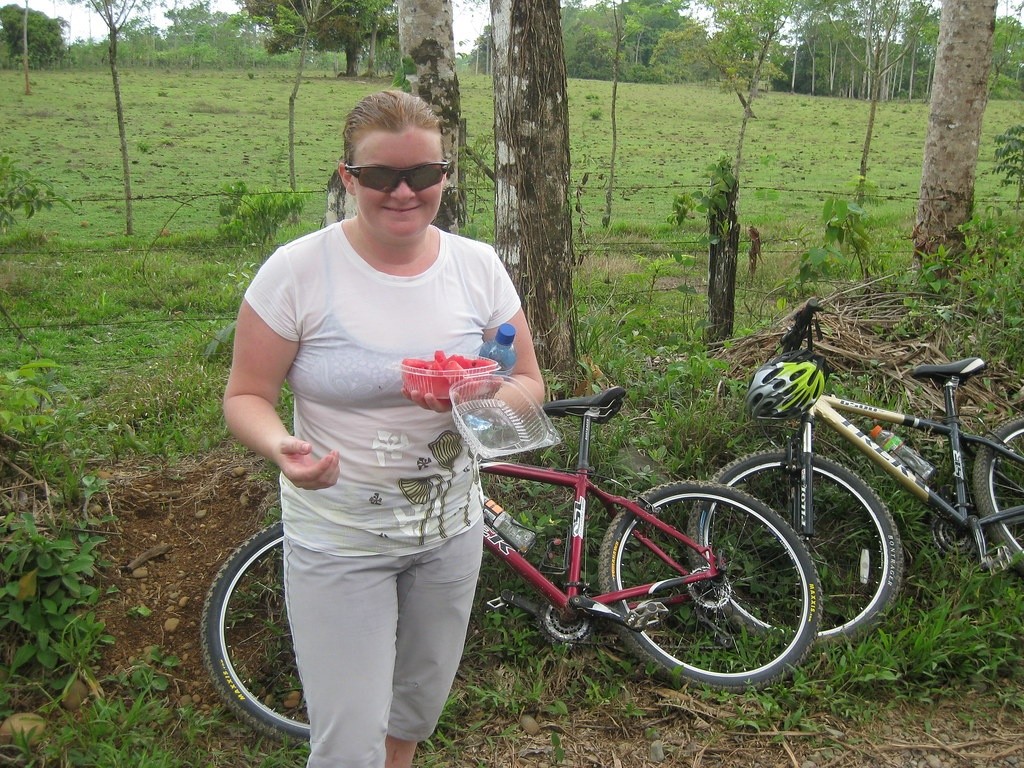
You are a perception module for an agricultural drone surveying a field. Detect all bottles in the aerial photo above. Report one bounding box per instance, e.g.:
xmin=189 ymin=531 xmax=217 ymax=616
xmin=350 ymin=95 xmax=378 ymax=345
xmin=476 ymin=323 xmax=517 ymax=378
xmin=871 ymin=424 xmax=935 ymax=483
xmin=482 ymin=494 xmax=536 ymax=555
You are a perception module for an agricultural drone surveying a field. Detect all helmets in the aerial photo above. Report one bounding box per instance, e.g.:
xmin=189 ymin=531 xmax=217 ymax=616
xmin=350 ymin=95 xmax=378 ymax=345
xmin=745 ymin=350 xmax=830 ymax=420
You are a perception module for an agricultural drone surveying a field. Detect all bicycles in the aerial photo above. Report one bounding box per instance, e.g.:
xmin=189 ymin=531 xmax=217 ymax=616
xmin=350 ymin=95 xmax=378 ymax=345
xmin=685 ymin=296 xmax=1024 ymax=655
xmin=197 ymin=381 xmax=823 ymax=750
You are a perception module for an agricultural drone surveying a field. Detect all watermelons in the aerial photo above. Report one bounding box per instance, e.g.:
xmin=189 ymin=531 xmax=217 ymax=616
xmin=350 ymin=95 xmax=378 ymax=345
xmin=402 ymin=350 xmax=497 ymax=399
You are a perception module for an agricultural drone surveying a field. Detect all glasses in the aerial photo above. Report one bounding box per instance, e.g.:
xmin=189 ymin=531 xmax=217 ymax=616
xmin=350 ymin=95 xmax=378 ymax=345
xmin=344 ymin=158 xmax=450 ymax=193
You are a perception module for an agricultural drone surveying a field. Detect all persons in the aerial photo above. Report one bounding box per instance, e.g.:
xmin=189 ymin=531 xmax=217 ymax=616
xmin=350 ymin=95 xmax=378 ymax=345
xmin=222 ymin=89 xmax=545 ymax=768
